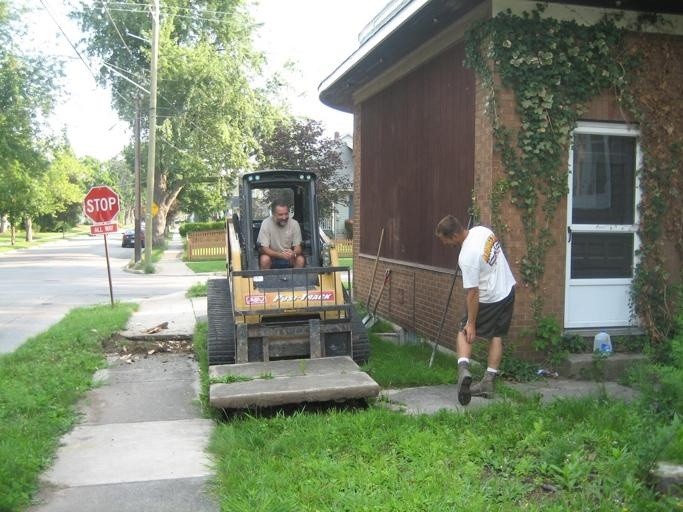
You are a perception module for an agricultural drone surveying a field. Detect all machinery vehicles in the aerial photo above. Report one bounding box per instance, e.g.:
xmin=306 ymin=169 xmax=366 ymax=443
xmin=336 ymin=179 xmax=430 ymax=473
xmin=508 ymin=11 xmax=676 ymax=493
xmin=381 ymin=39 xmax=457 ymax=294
xmin=206 ymin=170 xmax=371 ymax=367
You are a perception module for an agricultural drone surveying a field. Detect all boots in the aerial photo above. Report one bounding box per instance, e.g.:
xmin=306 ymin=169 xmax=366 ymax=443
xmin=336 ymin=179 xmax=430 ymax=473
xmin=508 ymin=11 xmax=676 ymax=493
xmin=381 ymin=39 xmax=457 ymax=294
xmin=470 ymin=371 xmax=496 ymax=399
xmin=457 ymin=362 xmax=471 ymax=405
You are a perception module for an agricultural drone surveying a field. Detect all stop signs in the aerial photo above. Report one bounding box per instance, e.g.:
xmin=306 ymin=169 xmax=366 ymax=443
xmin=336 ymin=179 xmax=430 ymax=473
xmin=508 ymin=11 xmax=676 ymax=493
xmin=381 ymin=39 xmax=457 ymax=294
xmin=82 ymin=185 xmax=120 ymax=224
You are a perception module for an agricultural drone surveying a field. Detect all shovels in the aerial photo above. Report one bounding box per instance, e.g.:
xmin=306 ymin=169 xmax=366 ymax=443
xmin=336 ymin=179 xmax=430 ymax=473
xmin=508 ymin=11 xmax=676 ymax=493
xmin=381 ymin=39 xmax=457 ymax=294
xmin=365 ymin=268 xmax=391 ymax=329
xmin=362 ymin=228 xmax=385 ymax=325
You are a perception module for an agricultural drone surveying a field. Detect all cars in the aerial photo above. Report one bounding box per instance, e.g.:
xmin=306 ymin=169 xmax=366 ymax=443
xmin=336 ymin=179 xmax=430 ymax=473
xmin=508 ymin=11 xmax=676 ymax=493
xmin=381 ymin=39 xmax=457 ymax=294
xmin=120 ymin=228 xmax=145 ymax=248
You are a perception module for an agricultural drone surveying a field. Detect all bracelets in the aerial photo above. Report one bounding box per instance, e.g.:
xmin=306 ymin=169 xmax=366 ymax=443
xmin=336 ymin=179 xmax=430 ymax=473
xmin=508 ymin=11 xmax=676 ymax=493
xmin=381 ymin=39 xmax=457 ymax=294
xmin=293 ymin=252 xmax=296 ymax=259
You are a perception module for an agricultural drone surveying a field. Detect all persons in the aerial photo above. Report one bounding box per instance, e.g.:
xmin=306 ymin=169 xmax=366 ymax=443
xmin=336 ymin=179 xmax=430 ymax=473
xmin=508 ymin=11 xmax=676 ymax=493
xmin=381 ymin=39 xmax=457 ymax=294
xmin=434 ymin=216 xmax=516 ymax=406
xmin=256 ymin=200 xmax=307 ymax=269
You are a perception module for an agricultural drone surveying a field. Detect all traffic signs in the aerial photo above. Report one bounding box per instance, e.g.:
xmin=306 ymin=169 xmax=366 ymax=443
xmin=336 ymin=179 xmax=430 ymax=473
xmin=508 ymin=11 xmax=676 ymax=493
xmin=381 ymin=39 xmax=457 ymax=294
xmin=90 ymin=224 xmax=117 ymax=234
